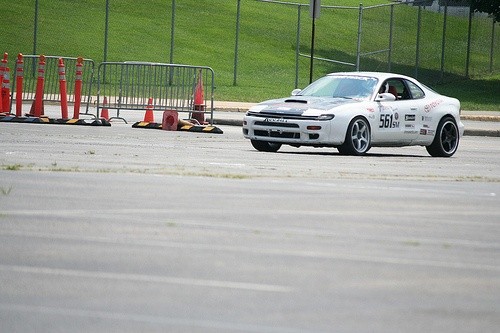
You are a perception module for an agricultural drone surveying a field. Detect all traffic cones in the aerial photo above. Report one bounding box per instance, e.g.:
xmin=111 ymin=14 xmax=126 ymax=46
xmin=144 ymin=97 xmax=154 ymax=123
xmin=100 ymin=96 xmax=110 ymax=122
xmin=162 ymin=110 xmax=178 ymax=132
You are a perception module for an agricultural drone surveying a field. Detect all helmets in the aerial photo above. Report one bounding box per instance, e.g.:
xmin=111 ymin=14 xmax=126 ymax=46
xmin=373 ymin=80 xmax=389 ymax=93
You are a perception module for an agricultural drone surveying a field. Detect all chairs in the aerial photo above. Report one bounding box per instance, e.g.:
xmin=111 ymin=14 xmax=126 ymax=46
xmin=388 ymin=86 xmax=398 ymax=100
xmin=401 ymin=87 xmax=409 ymax=99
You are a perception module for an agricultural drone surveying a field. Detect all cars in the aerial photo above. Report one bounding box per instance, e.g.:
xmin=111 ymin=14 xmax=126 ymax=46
xmin=242 ymin=70 xmax=465 ymax=158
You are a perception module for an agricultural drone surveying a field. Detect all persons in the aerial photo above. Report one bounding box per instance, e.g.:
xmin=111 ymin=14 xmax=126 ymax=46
xmin=378 ymin=81 xmax=389 ymax=98
